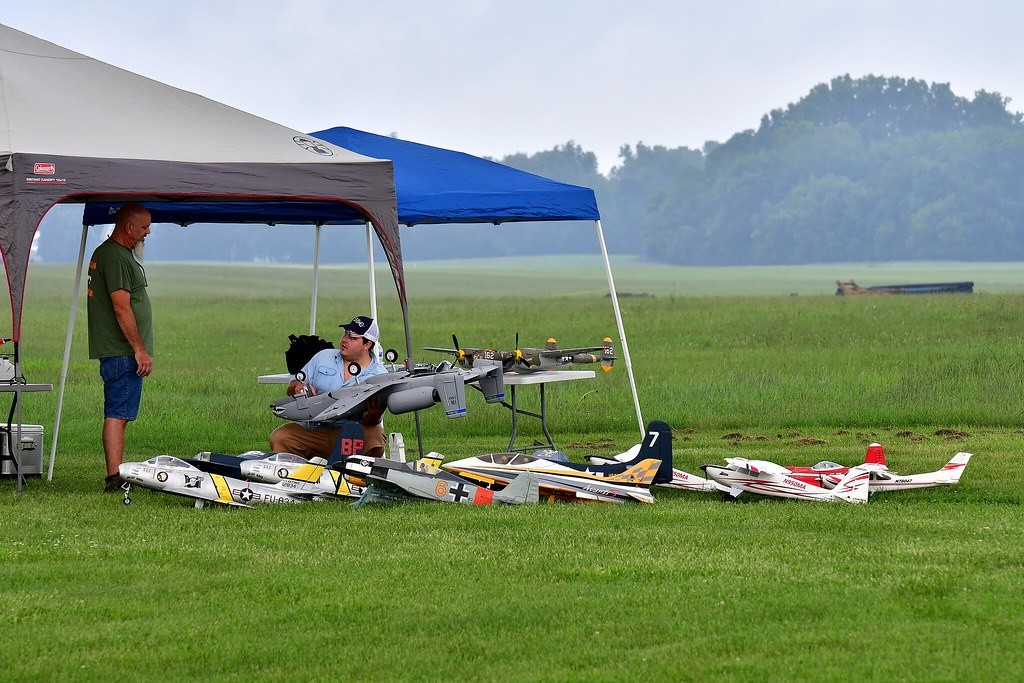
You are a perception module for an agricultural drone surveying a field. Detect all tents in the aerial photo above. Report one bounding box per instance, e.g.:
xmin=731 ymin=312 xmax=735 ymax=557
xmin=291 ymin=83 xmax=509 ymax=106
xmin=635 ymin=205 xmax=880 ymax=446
xmin=0 ymin=26 xmax=437 ymax=482
xmin=44 ymin=121 xmax=652 ymax=490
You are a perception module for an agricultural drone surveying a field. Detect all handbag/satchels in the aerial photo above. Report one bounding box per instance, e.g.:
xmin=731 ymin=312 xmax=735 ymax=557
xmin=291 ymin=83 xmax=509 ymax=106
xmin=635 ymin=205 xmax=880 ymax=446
xmin=285 ymin=334 xmax=335 ymax=375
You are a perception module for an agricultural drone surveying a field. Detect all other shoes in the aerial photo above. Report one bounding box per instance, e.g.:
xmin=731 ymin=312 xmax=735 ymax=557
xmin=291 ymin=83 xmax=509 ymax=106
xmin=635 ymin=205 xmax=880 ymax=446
xmin=105 ymin=472 xmax=135 ymax=493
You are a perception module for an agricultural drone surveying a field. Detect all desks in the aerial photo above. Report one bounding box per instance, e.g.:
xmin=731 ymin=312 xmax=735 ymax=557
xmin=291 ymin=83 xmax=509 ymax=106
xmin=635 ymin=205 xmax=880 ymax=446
xmin=0 ymin=383 xmax=54 ymax=494
xmin=257 ymin=370 xmax=596 ymax=453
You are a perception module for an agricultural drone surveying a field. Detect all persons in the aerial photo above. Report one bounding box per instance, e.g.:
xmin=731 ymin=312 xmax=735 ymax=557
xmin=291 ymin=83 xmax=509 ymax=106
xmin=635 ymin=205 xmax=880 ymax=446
xmin=86 ymin=201 xmax=154 ymax=493
xmin=270 ymin=315 xmax=389 ymax=463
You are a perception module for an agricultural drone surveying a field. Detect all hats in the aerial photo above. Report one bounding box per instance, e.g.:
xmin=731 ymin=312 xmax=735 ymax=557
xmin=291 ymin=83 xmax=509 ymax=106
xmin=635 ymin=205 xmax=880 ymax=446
xmin=339 ymin=315 xmax=380 ymax=343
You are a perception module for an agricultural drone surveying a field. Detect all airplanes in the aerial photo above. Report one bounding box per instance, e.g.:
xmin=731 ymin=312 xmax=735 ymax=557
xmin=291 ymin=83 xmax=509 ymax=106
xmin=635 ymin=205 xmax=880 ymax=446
xmin=423 ymin=330 xmax=619 ymax=375
xmin=116 ymin=420 xmax=672 ymax=513
xmin=697 ymin=454 xmax=871 ymax=503
xmin=270 ymin=349 xmax=504 ymax=432
xmin=585 ymin=444 xmax=744 ymax=500
xmin=815 ymin=442 xmax=974 ymax=494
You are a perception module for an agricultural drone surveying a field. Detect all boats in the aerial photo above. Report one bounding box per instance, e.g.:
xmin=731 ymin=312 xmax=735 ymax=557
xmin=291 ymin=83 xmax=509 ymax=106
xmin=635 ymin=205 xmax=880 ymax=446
xmin=836 ymin=280 xmax=974 ymax=295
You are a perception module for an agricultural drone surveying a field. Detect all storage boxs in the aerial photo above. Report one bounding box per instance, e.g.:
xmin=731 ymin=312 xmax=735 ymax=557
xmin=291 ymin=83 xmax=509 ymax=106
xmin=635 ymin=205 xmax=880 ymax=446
xmin=0 ymin=423 xmax=44 ymax=475
